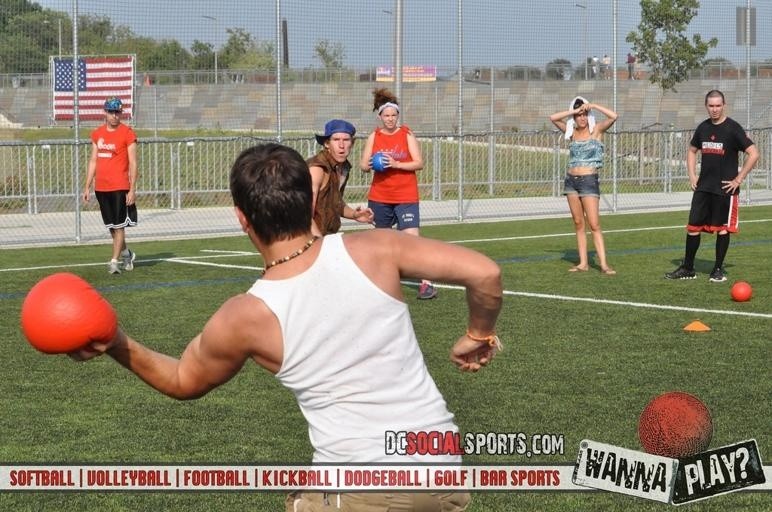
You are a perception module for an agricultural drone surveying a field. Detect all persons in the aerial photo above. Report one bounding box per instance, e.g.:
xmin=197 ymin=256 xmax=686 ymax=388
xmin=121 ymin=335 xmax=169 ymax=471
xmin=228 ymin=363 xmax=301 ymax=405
xmin=358 ymin=85 xmax=439 ymax=300
xmin=589 ymin=55 xmax=599 ymax=81
xmin=303 ymin=117 xmax=376 ymax=242
xmin=67 ymin=142 xmax=507 ymax=511
xmin=634 ymin=54 xmax=641 ymax=80
xmin=546 ymin=94 xmax=620 ymax=278
xmin=625 ymin=53 xmax=635 ymax=82
xmin=662 ymin=88 xmax=761 ymax=284
xmin=601 ymin=54 xmax=612 ymax=80
xmin=82 ymin=95 xmax=140 ymax=279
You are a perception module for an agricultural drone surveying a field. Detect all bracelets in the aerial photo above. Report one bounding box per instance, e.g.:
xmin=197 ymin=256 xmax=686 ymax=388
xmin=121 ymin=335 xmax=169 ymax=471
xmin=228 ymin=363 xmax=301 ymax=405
xmin=463 ymin=323 xmax=503 ymax=353
xmin=735 ymin=178 xmax=741 ymax=185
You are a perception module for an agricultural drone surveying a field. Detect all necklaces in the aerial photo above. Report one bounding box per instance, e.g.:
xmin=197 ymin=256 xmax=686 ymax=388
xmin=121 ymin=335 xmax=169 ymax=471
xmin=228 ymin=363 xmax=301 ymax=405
xmin=257 ymin=234 xmax=324 ymax=278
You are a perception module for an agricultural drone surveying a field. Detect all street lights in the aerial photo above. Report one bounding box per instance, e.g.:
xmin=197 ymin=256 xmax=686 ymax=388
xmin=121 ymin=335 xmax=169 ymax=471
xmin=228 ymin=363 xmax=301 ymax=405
xmin=574 ymin=2 xmax=589 ymax=81
xmin=202 ymin=15 xmax=218 ymax=84
xmin=43 ymin=18 xmax=62 ymax=60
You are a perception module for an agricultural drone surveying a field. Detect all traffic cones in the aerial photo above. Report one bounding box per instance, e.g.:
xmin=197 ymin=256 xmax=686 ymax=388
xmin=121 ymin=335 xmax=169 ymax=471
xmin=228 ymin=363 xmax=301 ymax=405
xmin=144 ymin=76 xmax=151 ymax=86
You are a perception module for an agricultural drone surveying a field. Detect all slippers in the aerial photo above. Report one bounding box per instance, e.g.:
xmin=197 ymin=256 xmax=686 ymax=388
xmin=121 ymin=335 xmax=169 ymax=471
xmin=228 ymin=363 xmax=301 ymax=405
xmin=600 ymin=267 xmax=616 ymax=274
xmin=567 ymin=266 xmax=590 ymax=273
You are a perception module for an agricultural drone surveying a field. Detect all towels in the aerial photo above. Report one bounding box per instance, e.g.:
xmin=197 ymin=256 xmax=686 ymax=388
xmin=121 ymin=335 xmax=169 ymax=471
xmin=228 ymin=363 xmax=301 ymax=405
xmin=565 ymin=96 xmax=595 ymax=139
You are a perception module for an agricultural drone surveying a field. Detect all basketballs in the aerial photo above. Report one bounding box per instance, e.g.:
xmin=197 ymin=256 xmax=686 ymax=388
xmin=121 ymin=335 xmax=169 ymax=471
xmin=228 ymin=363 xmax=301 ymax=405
xmin=639 ymin=391 xmax=713 ymax=458
xmin=731 ymin=282 xmax=752 ymax=301
xmin=371 ymin=153 xmax=389 ymax=173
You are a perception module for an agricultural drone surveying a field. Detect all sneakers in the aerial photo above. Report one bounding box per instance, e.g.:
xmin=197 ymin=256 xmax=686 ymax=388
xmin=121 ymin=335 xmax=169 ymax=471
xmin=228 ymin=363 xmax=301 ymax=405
xmin=664 ymin=266 xmax=697 ymax=280
xmin=417 ymin=284 xmax=437 ymax=299
xmin=708 ymin=270 xmax=727 ymax=282
xmin=108 ymin=262 xmax=121 ymax=275
xmin=119 ymin=249 xmax=136 ymax=271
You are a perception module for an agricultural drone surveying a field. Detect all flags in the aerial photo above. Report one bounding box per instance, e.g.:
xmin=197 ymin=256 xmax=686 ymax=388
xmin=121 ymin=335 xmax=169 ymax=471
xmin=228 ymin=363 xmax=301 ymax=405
xmin=50 ymin=56 xmax=137 ymax=123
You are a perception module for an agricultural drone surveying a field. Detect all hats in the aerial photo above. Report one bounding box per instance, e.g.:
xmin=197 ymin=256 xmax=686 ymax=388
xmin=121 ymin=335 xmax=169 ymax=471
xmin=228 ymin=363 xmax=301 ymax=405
xmin=103 ymin=98 xmax=123 ymax=112
xmin=315 ymin=120 xmax=356 ymax=145
